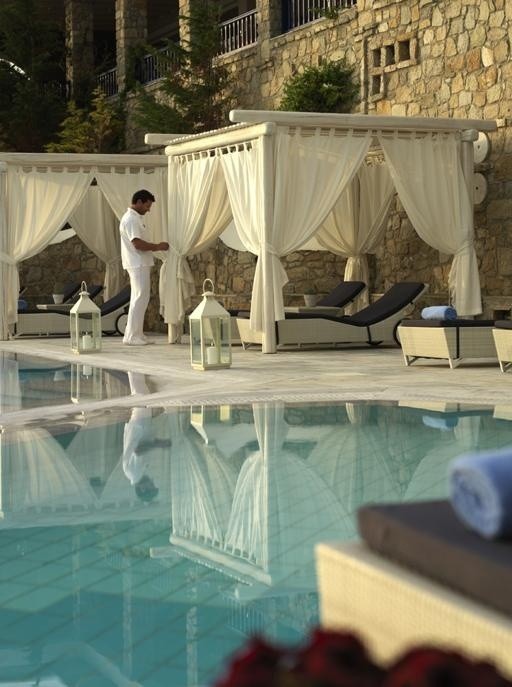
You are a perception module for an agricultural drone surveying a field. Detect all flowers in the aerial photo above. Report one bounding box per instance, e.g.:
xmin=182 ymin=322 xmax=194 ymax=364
xmin=208 ymin=626 xmax=511 ymax=686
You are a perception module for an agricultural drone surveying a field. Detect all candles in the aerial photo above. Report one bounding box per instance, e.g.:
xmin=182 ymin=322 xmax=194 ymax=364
xmin=207 ymin=342 xmax=219 ymax=364
xmin=83 ymin=331 xmax=91 ymax=349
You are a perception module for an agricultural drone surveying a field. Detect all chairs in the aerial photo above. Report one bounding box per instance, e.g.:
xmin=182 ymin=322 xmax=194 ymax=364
xmin=222 ymin=275 xmax=512 ymax=375
xmin=12 ymin=278 xmax=154 ymax=337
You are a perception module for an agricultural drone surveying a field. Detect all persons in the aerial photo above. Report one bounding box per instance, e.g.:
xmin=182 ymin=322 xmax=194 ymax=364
xmin=121 ymin=372 xmax=160 ymax=503
xmin=118 ymin=187 xmax=170 ymax=347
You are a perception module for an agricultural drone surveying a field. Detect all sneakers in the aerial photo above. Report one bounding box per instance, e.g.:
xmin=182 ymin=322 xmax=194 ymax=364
xmin=123 ymin=335 xmax=155 ymax=345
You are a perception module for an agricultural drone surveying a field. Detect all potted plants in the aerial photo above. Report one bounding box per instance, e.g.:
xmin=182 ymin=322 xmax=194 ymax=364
xmin=51 ymin=281 xmax=66 ymax=305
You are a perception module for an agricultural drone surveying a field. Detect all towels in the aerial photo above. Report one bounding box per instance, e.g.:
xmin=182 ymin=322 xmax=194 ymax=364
xmin=446 ymin=444 xmax=512 ymax=541
xmin=422 ymin=305 xmax=457 ymax=320
